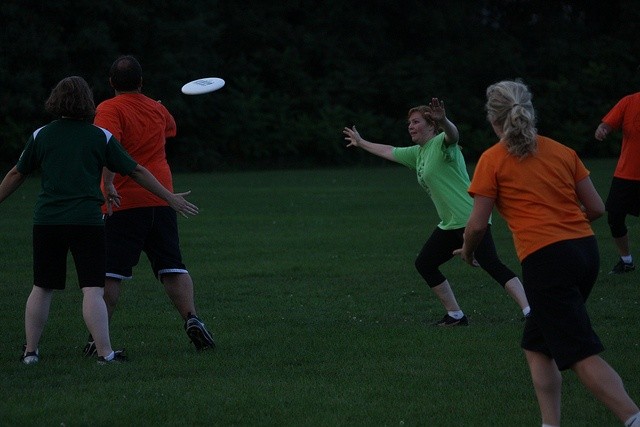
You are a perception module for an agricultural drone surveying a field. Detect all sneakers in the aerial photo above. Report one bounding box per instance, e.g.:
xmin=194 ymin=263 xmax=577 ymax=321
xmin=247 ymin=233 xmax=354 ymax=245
xmin=609 ymin=258 xmax=636 ymax=275
xmin=184 ymin=312 xmax=217 ymax=353
xmin=430 ymin=314 xmax=469 ymax=329
xmin=21 ymin=344 xmax=39 ymax=365
xmin=97 ymin=348 xmax=131 ymax=365
xmin=81 ymin=334 xmax=98 ymax=361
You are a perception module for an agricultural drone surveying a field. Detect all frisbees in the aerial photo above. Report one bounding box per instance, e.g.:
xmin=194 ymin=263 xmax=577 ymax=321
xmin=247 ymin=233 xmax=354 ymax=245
xmin=181 ymin=77 xmax=225 ymax=95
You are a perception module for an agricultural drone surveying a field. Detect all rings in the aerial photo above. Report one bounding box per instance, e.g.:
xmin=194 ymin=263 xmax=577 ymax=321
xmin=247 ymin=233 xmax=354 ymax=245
xmin=109 ymin=198 xmax=112 ymax=202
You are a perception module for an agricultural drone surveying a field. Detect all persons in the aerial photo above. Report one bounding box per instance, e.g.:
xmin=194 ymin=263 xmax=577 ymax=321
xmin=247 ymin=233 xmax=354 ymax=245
xmin=0 ymin=76 xmax=199 ymax=366
xmin=594 ymin=91 xmax=639 ymax=276
xmin=83 ymin=56 xmax=216 ymax=359
xmin=342 ymin=98 xmax=532 ymax=328
xmin=453 ymin=81 xmax=639 ymax=427
